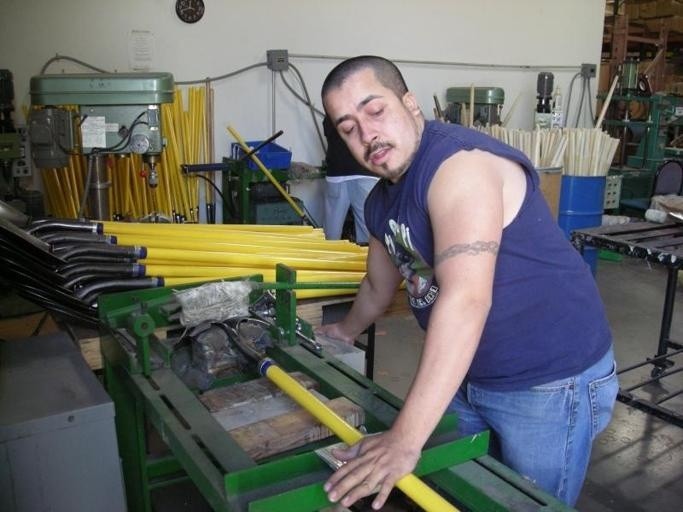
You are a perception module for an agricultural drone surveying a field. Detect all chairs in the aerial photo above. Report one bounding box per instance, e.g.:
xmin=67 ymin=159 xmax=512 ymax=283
xmin=617 ymin=160 xmax=683 ymax=216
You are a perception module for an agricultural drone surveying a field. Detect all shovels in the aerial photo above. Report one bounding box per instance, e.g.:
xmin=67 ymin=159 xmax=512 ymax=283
xmin=0 ymin=199 xmax=408 ymax=329
xmin=175 ymin=317 xmax=462 ymax=512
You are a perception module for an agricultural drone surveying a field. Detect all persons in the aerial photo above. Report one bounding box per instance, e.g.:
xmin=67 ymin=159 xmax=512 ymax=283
xmin=313 ymin=56 xmax=619 ymax=510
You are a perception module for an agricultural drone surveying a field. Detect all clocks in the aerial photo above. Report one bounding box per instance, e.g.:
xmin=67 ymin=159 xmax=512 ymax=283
xmin=176 ymin=0 xmax=205 ymax=23
